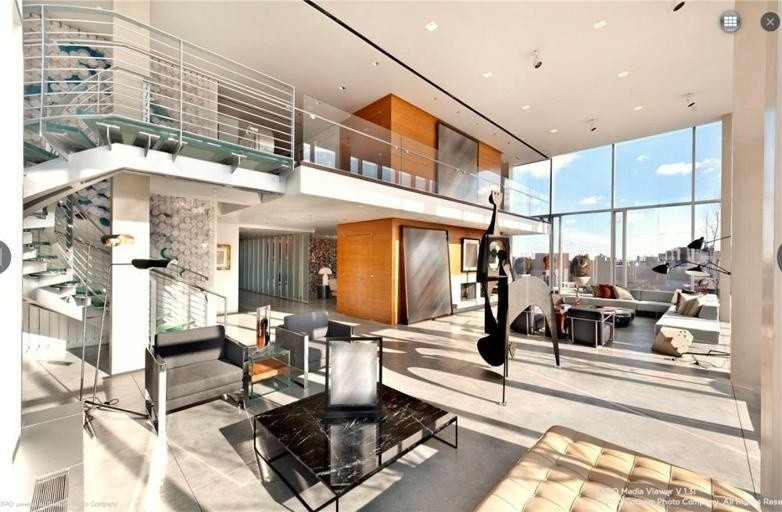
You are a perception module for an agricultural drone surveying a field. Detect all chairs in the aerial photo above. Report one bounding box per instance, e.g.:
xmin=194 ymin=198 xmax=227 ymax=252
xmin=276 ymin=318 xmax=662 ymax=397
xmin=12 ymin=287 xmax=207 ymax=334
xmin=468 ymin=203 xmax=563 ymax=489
xmin=565 ymin=307 xmax=615 ymax=347
xmin=143 ymin=309 xmax=387 ymax=436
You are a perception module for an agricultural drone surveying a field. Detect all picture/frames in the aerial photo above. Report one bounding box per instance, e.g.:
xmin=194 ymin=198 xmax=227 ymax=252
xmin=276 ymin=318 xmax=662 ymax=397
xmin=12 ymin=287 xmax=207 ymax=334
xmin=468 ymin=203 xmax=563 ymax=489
xmin=216 ymin=244 xmax=231 ymax=271
xmin=461 ymin=237 xmax=480 ymax=272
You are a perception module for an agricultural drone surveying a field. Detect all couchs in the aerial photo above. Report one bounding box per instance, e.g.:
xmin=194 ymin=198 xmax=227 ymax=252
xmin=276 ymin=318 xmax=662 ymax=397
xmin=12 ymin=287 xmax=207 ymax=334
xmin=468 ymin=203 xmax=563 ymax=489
xmin=565 ymin=285 xmax=720 ymax=344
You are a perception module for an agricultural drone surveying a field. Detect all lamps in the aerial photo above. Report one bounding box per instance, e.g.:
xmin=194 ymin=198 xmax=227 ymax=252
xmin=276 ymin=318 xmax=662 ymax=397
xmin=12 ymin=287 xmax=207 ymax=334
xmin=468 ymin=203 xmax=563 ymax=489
xmin=79 ymin=233 xmax=172 ymax=442
xmin=318 ymin=267 xmax=333 ymax=286
xmin=687 ymin=235 xmax=730 ymax=253
xmin=652 ymin=258 xmax=731 ymax=279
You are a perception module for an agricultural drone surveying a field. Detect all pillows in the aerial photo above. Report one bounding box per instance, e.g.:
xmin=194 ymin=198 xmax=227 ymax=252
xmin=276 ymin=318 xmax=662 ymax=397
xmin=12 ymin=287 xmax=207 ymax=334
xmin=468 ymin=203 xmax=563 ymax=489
xmin=591 ymin=283 xmax=634 ymax=301
xmin=670 ymin=288 xmax=707 ymax=317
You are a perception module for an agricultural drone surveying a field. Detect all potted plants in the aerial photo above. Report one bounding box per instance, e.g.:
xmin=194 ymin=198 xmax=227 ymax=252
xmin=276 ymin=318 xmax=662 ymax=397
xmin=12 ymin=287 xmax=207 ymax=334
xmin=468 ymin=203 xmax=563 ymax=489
xmin=569 ymin=253 xmax=592 ymax=286
xmin=514 ymin=257 xmax=532 ymax=280
